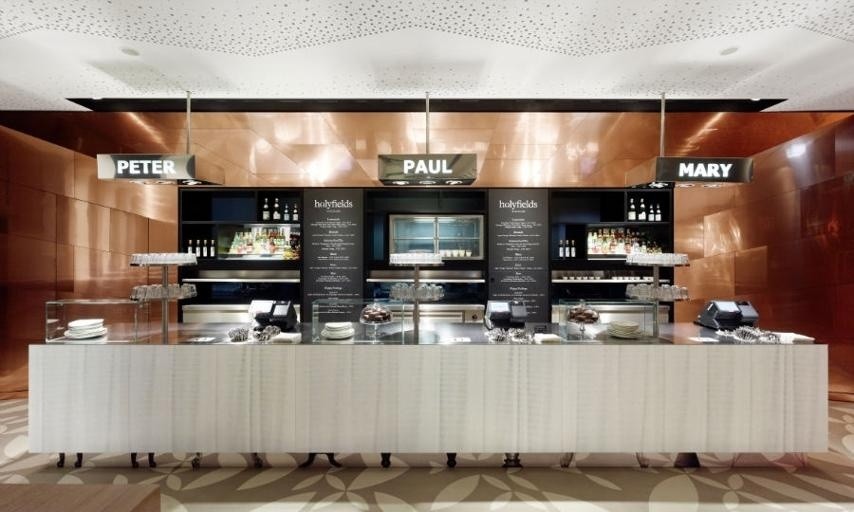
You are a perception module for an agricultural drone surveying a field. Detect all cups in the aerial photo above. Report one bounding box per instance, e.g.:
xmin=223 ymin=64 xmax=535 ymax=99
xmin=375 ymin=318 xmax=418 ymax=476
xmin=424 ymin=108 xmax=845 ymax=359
xmin=627 ymin=254 xmax=690 ymax=264
xmin=131 ymin=251 xmax=196 ymax=263
xmin=626 ymin=283 xmax=688 ymax=302
xmin=391 ymin=283 xmax=443 ymax=302
xmin=130 ymin=284 xmax=196 ymax=302
xmin=441 ymin=249 xmax=472 ymax=257
xmin=389 ymin=253 xmax=442 ymax=265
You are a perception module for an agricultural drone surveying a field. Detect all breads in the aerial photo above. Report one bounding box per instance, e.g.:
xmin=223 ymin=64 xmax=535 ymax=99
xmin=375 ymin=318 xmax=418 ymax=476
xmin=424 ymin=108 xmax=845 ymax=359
xmin=568 ymin=307 xmax=598 ymax=323
xmin=363 ymin=307 xmax=391 ymax=321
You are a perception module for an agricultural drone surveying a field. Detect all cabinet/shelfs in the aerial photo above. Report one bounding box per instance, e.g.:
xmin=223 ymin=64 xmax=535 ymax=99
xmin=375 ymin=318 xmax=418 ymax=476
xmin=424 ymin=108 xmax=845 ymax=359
xmin=178 ymin=187 xmax=302 ymax=326
xmin=547 ymin=187 xmax=674 ymax=325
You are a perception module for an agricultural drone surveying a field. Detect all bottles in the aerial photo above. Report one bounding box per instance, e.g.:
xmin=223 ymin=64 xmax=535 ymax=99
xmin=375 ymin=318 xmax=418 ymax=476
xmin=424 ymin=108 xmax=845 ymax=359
xmin=230 ymin=228 xmax=301 ymax=254
xmin=188 ymin=240 xmax=216 ymax=257
xmin=558 ymin=240 xmax=576 ymax=258
xmin=263 ymin=197 xmax=299 ymax=223
xmin=628 ymin=198 xmax=663 ymax=222
xmin=586 ymin=226 xmax=664 ymax=258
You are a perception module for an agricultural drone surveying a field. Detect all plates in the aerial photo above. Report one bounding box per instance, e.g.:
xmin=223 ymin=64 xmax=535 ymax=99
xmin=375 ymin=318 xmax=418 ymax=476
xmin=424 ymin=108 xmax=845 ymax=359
xmin=319 ymin=322 xmax=354 ymax=340
xmin=62 ymin=319 xmax=109 ymax=341
xmin=607 ymin=321 xmax=642 ymax=340
